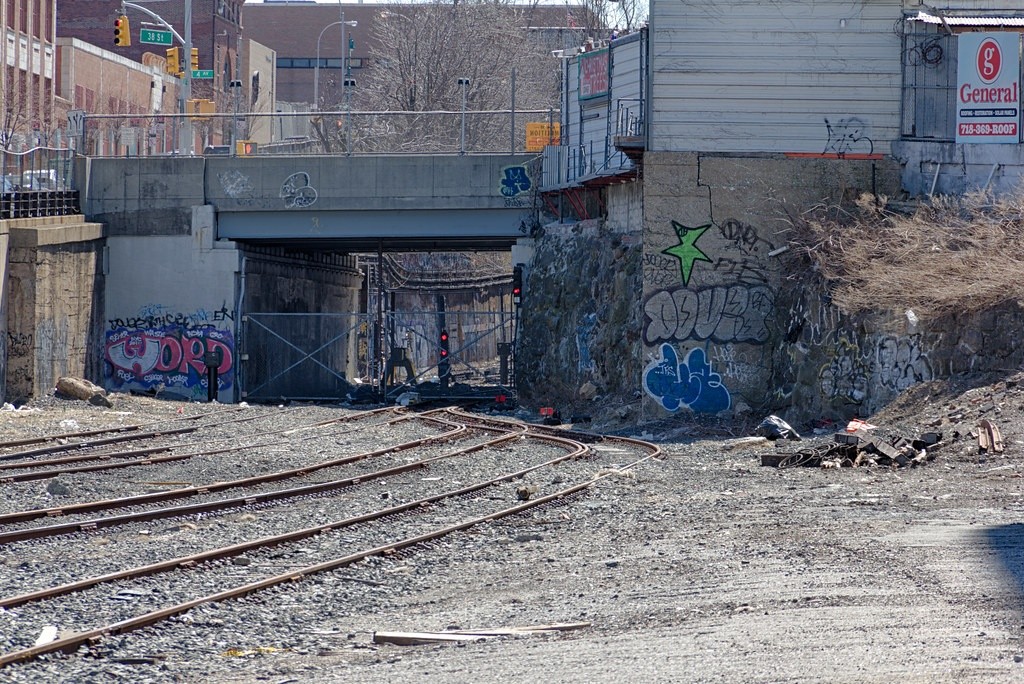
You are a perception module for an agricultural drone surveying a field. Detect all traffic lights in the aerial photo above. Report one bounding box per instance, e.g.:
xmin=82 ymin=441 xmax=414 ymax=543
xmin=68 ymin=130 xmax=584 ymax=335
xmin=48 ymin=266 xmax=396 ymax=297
xmin=512 ymin=265 xmax=522 ymax=305
xmin=235 ymin=139 xmax=257 ymax=154
xmin=440 ymin=330 xmax=449 ymax=359
xmin=166 ymin=47 xmax=179 ymax=73
xmin=191 ymin=48 xmax=198 ymax=71
xmin=114 ymin=16 xmax=127 ymax=47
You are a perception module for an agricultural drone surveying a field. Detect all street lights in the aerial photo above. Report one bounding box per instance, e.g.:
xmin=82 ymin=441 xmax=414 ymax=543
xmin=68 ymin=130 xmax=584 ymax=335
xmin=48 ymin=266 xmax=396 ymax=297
xmin=313 ymin=20 xmax=358 ymax=112
xmin=344 ymin=79 xmax=357 ymax=153
xmin=229 ymin=80 xmax=242 ymax=156
xmin=457 ymin=77 xmax=471 ymax=151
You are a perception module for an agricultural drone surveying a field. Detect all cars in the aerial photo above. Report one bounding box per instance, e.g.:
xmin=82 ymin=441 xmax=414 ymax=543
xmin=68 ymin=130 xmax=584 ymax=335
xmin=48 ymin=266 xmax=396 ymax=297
xmin=0 ymin=169 xmax=66 ymax=194
xmin=204 ymin=145 xmax=237 ymax=155
